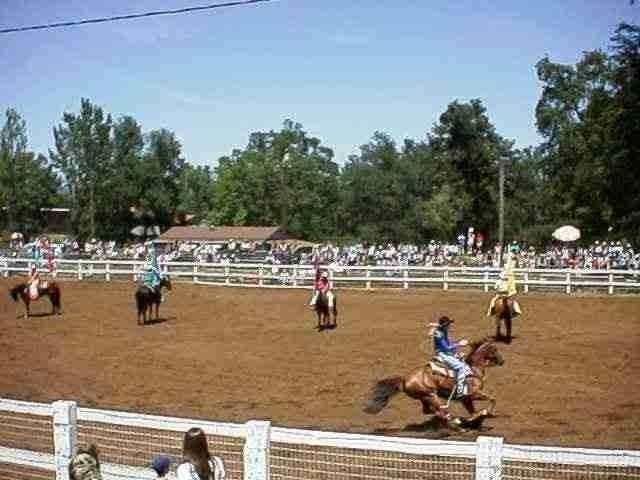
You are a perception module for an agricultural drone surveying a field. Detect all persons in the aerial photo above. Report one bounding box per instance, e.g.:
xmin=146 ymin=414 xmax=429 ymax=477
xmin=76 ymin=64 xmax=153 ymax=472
xmin=29 ymin=265 xmax=40 ymax=300
xmin=68 ymin=443 xmax=102 ymax=480
xmin=143 ymin=265 xmax=159 ymax=295
xmin=177 ymin=428 xmax=225 ymax=480
xmin=433 ymin=317 xmax=468 ymax=392
xmin=132 ymin=457 xmax=170 ymax=479
xmin=0 ymin=222 xmax=640 ymax=273
xmin=311 ymin=272 xmax=335 ymax=311
xmin=485 ymin=272 xmax=522 ymax=318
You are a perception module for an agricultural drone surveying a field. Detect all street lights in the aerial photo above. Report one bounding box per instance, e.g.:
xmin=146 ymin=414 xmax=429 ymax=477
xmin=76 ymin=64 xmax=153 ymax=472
xmin=491 ymin=155 xmax=515 ymax=245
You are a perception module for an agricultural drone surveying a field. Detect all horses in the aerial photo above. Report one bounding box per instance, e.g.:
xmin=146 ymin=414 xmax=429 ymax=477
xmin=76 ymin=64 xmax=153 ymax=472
xmin=8 ymin=281 xmax=61 ymax=319
xmin=135 ymin=275 xmax=172 ymax=325
xmin=494 ymin=295 xmax=520 ymax=344
xmin=363 ymin=334 xmax=505 ymax=429
xmin=315 ymin=287 xmax=337 ymax=329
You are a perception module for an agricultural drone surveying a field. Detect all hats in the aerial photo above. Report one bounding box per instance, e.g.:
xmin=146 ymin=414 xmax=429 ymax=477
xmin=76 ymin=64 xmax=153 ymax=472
xmin=154 ymin=456 xmax=168 ymax=474
xmin=439 ymin=316 xmax=453 ymax=327
